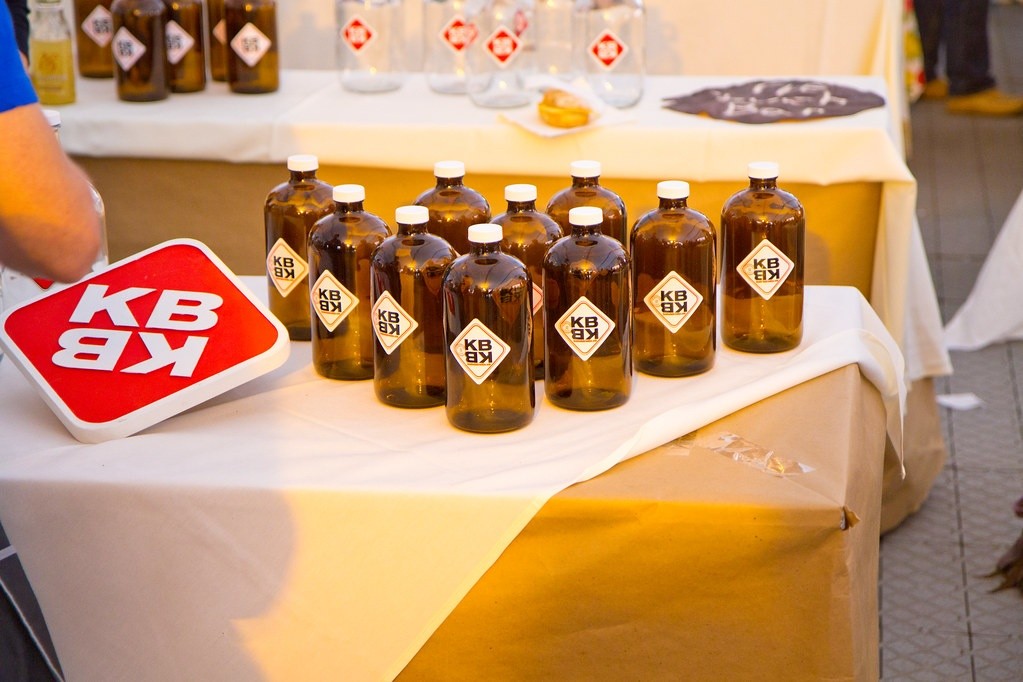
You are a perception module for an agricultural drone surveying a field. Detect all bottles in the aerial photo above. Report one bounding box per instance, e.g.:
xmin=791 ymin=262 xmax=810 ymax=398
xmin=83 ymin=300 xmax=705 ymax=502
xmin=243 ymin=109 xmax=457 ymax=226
xmin=720 ymin=162 xmax=805 ymax=354
xmin=74 ymin=1 xmax=118 ymax=78
xmin=575 ymin=0 xmax=646 ymax=107
xmin=492 ymin=184 xmax=565 ymax=381
xmin=207 ymin=0 xmax=229 ymax=82
xmin=410 ymin=161 xmax=490 ymax=256
xmin=442 ymin=223 xmax=536 ymax=434
xmin=227 ymin=0 xmax=279 ymax=93
xmin=370 ymin=204 xmax=462 ymax=410
xmin=544 ymin=162 xmax=628 ymax=251
xmin=542 ymin=206 xmax=632 ymax=412
xmin=631 ymin=180 xmax=716 ymax=377
xmin=112 ymin=1 xmax=168 ymax=102
xmin=463 ymin=0 xmax=536 ymax=107
xmin=307 ymin=183 xmax=393 ymax=381
xmin=335 ymin=0 xmax=403 ymax=92
xmin=422 ymin=0 xmax=494 ymax=94
xmin=27 ymin=0 xmax=78 ymax=104
xmin=10 ymin=109 xmax=108 ymax=291
xmin=163 ymin=1 xmax=206 ymax=93
xmin=264 ymin=154 xmax=336 ymax=341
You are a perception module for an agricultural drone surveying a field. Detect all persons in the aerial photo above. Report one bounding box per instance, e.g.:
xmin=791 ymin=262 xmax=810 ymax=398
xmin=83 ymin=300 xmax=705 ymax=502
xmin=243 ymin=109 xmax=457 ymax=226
xmin=0 ymin=0 xmax=105 ymax=283
xmin=912 ymin=0 xmax=1023 ymax=117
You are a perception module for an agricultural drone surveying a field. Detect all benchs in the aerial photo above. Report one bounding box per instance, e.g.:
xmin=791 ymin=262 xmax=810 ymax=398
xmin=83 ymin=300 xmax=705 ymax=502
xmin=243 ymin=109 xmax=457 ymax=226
xmin=274 ymin=2 xmax=918 ymax=138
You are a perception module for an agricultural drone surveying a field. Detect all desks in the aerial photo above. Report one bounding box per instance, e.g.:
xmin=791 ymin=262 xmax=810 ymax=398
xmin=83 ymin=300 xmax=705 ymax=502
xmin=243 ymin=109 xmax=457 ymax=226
xmin=34 ymin=67 xmax=956 ymax=539
xmin=0 ymin=269 xmax=908 ymax=682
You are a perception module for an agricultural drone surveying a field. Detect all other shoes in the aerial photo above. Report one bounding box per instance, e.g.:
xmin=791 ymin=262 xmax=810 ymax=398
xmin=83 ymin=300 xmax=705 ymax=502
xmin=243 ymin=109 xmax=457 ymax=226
xmin=941 ymin=86 xmax=1023 ymax=118
xmin=920 ymin=77 xmax=950 ymax=104
